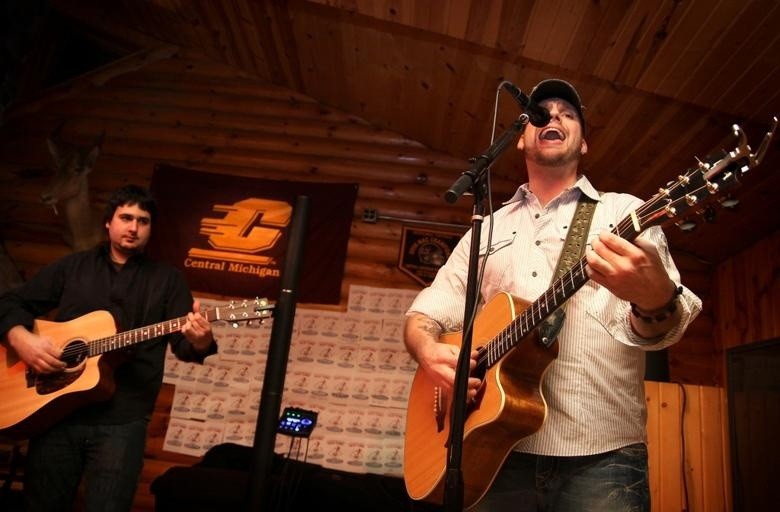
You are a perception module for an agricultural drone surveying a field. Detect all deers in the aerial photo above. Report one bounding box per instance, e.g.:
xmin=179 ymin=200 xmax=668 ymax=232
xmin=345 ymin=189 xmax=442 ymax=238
xmin=38 ymin=123 xmax=105 ymax=255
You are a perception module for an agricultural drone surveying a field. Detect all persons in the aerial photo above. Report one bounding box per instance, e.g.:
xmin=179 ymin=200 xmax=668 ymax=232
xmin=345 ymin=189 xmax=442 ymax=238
xmin=419 ymin=244 xmax=439 ymax=264
xmin=402 ymin=77 xmax=705 ymax=512
xmin=0 ymin=181 xmax=223 ymax=512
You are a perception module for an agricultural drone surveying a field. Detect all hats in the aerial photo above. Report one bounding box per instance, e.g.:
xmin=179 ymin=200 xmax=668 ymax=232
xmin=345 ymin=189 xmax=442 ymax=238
xmin=530 ymin=79 xmax=586 ymax=135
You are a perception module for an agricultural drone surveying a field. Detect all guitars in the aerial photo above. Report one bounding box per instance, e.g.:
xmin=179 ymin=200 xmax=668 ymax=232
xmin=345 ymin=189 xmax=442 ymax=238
xmin=404 ymin=116 xmax=778 ymax=512
xmin=0 ymin=296 xmax=276 ymax=440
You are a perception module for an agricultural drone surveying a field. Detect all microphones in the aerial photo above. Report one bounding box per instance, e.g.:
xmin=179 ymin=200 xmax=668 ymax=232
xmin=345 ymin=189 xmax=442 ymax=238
xmin=504 ymin=82 xmax=551 ymax=128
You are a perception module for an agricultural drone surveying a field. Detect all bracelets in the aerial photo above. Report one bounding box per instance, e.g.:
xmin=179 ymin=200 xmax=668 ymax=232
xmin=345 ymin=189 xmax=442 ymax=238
xmin=630 ymin=279 xmax=683 ymax=325
